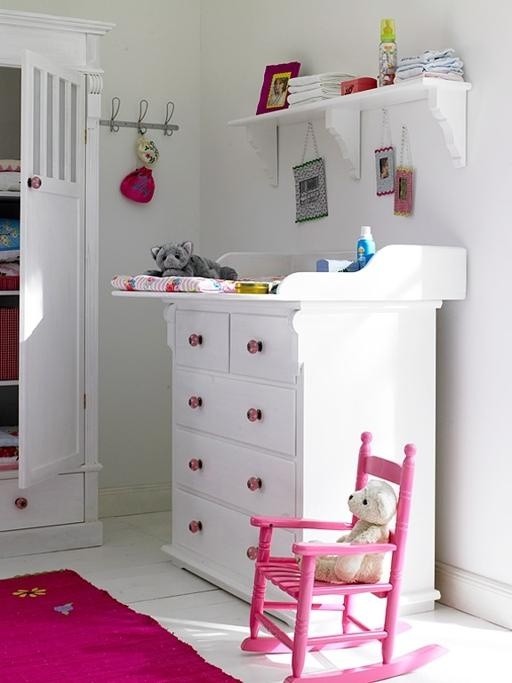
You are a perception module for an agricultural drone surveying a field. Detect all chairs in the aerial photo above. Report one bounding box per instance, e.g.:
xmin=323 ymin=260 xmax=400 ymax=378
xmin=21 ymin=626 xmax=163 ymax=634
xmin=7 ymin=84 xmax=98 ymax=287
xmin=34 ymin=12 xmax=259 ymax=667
xmin=241 ymin=433 xmax=434 ymax=683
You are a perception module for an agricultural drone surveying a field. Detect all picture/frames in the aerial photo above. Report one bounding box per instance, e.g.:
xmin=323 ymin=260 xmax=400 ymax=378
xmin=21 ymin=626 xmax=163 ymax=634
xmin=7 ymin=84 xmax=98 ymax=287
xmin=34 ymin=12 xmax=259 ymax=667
xmin=256 ymin=62 xmax=300 ymax=114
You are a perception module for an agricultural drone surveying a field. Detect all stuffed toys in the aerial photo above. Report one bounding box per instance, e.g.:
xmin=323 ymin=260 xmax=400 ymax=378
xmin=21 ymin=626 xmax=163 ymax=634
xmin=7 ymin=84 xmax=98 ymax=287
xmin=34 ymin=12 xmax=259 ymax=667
xmin=141 ymin=239 xmax=238 ymax=282
xmin=295 ymin=478 xmax=397 ymax=585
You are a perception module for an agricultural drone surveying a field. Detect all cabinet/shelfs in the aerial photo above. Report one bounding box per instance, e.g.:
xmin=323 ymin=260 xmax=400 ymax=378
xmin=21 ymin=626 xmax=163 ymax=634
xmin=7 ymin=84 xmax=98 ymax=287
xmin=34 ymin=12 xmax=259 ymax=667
xmin=112 ymin=245 xmax=466 ymax=631
xmin=2 ymin=8 xmax=115 ymax=561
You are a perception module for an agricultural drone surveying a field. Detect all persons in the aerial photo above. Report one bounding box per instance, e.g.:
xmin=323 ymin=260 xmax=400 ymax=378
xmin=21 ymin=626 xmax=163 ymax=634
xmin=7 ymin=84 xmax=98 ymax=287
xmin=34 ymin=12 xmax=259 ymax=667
xmin=380 ymin=157 xmax=388 ymax=179
xmin=270 ymin=76 xmax=288 ymax=105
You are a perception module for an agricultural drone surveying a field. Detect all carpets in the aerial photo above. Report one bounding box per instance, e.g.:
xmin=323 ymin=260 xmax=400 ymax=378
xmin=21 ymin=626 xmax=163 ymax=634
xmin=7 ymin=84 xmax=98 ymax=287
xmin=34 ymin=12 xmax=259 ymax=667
xmin=0 ymin=569 xmax=242 ymax=683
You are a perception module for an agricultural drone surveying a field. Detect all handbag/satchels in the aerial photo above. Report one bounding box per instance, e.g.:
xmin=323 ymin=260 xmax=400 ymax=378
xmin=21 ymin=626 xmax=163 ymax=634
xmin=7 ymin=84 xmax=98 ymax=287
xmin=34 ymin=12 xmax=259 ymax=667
xmin=121 ymin=167 xmax=154 ymax=203
xmin=136 ymin=136 xmax=160 ymax=166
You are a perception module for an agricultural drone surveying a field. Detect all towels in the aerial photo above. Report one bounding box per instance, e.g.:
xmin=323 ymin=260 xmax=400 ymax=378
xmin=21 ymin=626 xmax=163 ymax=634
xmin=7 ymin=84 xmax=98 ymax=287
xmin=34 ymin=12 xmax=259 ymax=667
xmin=285 ymin=72 xmax=354 ymax=107
xmin=394 ymin=48 xmax=464 ymax=81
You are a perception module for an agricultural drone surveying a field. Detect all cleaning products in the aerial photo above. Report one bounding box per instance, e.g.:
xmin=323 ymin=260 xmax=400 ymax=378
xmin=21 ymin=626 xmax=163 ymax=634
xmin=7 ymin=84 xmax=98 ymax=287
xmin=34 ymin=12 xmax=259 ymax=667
xmin=356 ymin=225 xmax=374 ymax=267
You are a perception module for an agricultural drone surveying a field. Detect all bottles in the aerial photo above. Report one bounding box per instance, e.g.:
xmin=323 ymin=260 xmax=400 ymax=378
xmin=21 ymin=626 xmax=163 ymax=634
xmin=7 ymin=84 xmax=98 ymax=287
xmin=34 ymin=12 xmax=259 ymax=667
xmin=378 ymin=18 xmax=398 ymax=87
